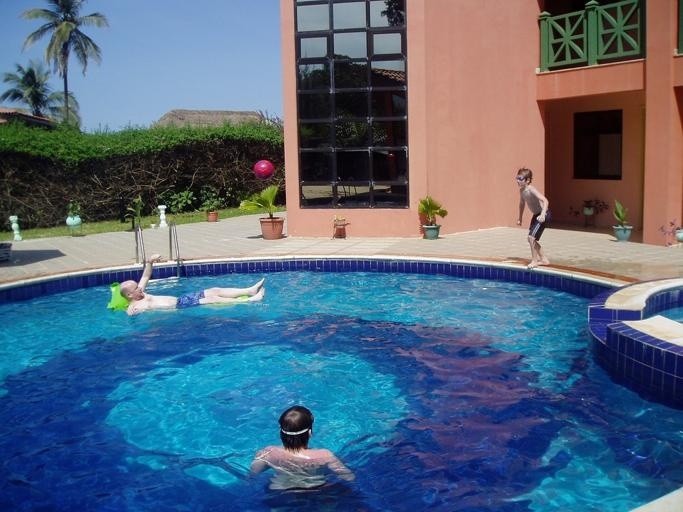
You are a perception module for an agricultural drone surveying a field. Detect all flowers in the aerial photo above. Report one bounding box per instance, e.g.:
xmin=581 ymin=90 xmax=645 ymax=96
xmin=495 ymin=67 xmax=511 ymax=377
xmin=658 ymin=218 xmax=683 ymax=247
xmin=566 ymin=198 xmax=609 ymax=217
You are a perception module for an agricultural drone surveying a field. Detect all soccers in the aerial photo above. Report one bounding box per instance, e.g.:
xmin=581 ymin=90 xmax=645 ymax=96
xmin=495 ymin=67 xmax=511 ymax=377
xmin=254 ymin=160 xmax=274 ymax=179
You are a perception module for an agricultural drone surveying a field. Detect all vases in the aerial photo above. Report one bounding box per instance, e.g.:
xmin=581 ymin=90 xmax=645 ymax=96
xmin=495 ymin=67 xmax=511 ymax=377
xmin=675 ymin=230 xmax=683 ymax=242
xmin=582 ymin=206 xmax=594 ymax=216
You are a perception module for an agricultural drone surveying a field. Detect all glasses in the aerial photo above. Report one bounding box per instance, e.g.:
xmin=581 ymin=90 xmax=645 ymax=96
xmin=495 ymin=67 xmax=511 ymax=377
xmin=516 ymin=176 xmax=525 ymax=181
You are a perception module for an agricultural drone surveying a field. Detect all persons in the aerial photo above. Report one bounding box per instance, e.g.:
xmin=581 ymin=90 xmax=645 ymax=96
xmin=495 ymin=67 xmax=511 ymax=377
xmin=246 ymin=405 xmax=358 ymax=495
xmin=513 ymin=166 xmax=553 ymax=271
xmin=117 ymin=253 xmax=267 ymax=316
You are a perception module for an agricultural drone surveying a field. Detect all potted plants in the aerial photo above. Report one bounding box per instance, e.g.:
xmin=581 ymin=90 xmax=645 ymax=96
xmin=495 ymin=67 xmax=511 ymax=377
xmin=64 ymin=199 xmax=81 ymax=229
xmin=198 ymin=194 xmax=222 ymax=223
xmin=237 ymin=184 xmax=284 ymax=240
xmin=611 ymin=199 xmax=633 ymax=242
xmin=418 ymin=193 xmax=448 ymax=240
